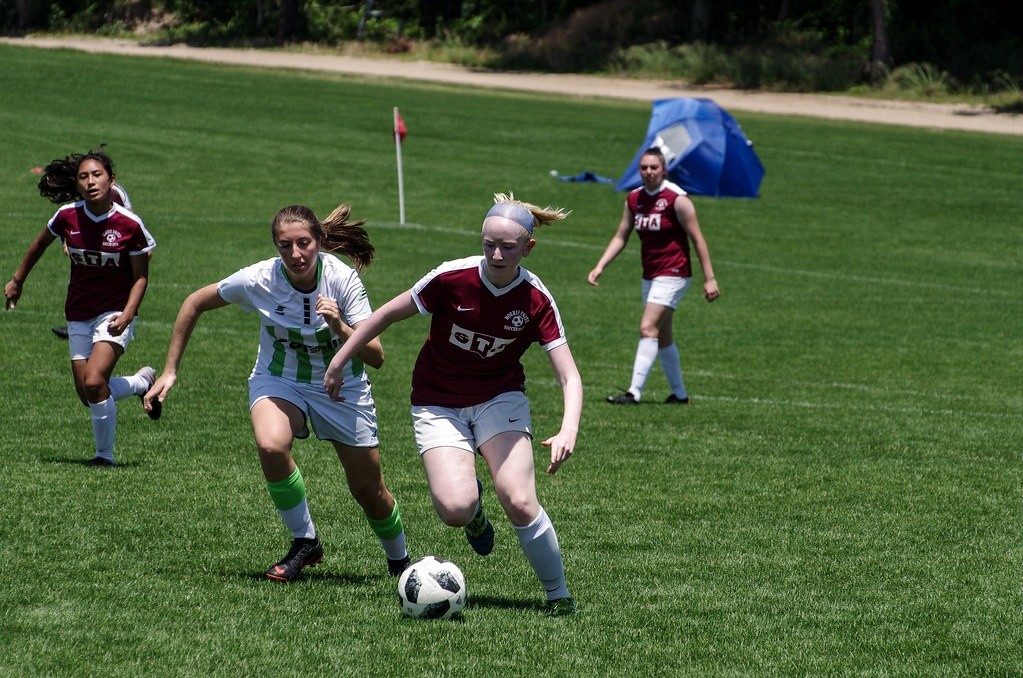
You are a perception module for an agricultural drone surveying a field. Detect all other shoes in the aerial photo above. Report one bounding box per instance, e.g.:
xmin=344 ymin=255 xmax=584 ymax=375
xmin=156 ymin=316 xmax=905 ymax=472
xmin=52 ymin=326 xmax=69 ymax=340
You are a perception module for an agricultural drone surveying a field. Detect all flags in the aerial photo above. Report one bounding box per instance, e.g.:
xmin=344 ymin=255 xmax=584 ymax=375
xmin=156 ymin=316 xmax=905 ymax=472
xmin=392 ymin=113 xmax=407 ymax=142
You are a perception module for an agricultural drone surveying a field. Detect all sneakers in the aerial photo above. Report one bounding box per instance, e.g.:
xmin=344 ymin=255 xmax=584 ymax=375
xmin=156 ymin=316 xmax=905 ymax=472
xmin=605 ymin=387 xmax=641 ymax=404
xmin=548 ymin=597 xmax=575 ymax=617
xmin=386 ymin=554 xmax=411 ymax=576
xmin=464 ymin=479 xmax=496 ymax=556
xmin=662 ymin=393 xmax=691 ymax=405
xmin=133 ymin=366 xmax=162 ymax=420
xmin=266 ymin=535 xmax=324 ymax=580
xmin=83 ymin=457 xmax=112 ymax=466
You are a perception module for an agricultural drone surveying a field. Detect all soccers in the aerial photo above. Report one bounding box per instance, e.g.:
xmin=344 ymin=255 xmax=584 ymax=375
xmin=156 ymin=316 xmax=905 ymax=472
xmin=397 ymin=555 xmax=467 ymax=622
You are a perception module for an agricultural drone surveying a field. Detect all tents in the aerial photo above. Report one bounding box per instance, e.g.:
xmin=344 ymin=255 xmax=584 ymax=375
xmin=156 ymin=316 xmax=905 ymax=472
xmin=616 ymin=95 xmax=766 ymax=197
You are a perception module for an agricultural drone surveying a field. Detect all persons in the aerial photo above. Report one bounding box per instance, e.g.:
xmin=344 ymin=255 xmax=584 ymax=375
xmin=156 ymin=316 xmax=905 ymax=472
xmin=5 ymin=150 xmax=163 ymax=467
xmin=143 ymin=204 xmax=410 ymax=582
xmin=322 ymin=192 xmax=584 ymax=614
xmin=588 ymin=147 xmax=720 ymax=406
